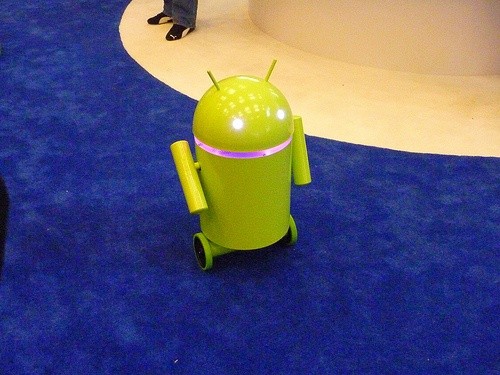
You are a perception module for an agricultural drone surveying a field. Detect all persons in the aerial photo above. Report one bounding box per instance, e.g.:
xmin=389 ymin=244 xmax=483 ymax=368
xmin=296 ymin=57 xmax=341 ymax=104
xmin=146 ymin=0 xmax=198 ymax=41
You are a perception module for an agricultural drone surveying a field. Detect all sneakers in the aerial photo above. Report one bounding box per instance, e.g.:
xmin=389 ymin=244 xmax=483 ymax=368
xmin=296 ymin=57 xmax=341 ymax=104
xmin=147 ymin=12 xmax=174 ymax=25
xmin=166 ymin=25 xmax=196 ymax=41
